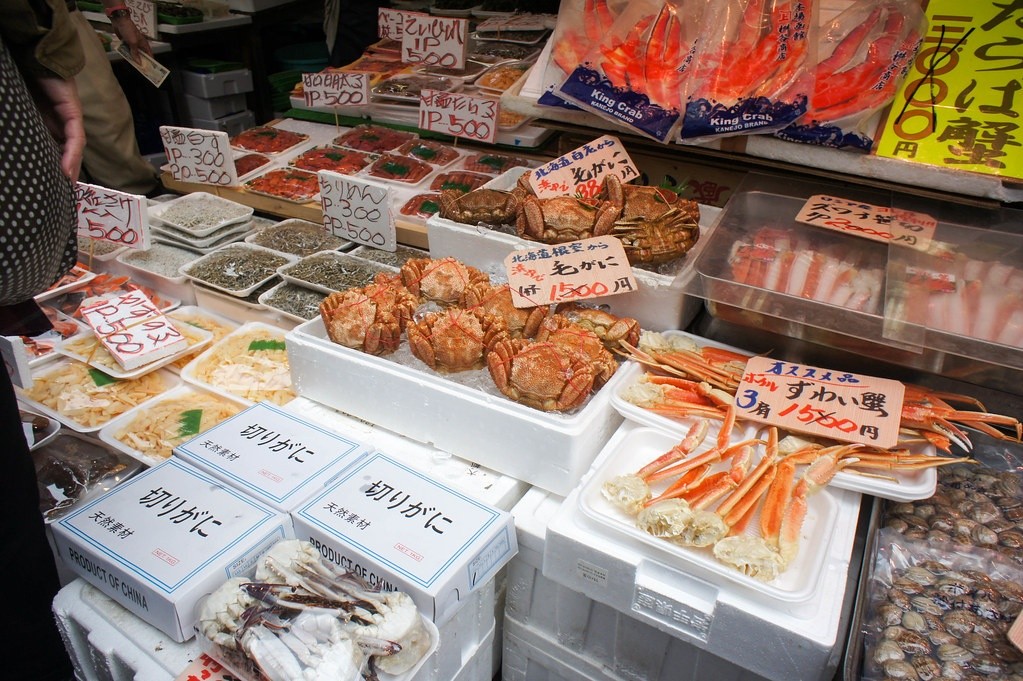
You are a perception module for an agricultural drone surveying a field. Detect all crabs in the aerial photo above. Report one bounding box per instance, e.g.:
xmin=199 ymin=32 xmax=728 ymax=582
xmin=19 ymin=261 xmax=171 ymax=352
xmin=537 ymin=0 xmax=924 ymax=144
xmin=196 ymin=538 xmax=429 ymax=680
xmin=319 ymin=174 xmax=1023 ymax=581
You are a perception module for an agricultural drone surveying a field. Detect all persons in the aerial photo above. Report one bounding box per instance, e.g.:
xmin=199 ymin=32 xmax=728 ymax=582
xmin=56 ymin=3 xmax=160 ymax=200
xmin=0 ymin=0 xmax=86 ymax=681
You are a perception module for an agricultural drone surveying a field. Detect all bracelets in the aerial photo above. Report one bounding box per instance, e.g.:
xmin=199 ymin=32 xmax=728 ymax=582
xmin=103 ymin=5 xmax=132 ymax=20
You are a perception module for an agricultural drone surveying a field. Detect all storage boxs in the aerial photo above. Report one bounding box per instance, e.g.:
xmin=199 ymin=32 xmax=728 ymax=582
xmin=186 ymin=110 xmax=257 ymax=136
xmin=287 ymin=294 xmax=645 ymax=498
xmin=184 ymin=92 xmax=248 ymax=120
xmin=424 ymin=165 xmax=725 ymax=331
xmin=500 ymin=483 xmax=865 ymax=680
xmin=47 ymin=579 xmax=495 ymax=681
xmin=540 ymin=417 xmax=862 ymax=681
xmin=181 ymin=68 xmax=255 ymax=99
